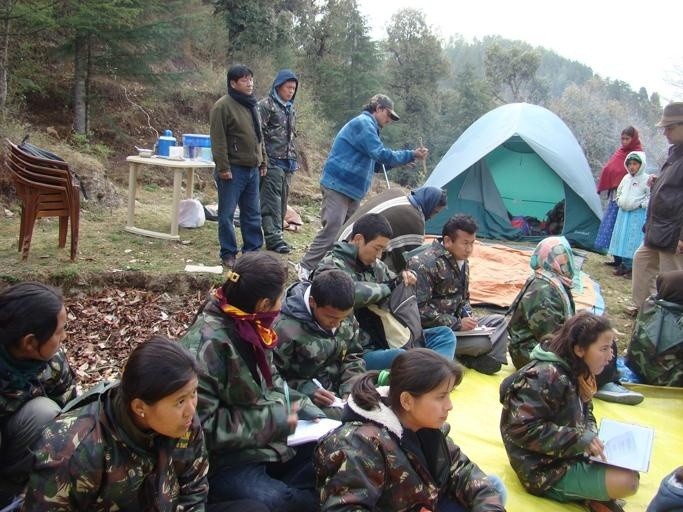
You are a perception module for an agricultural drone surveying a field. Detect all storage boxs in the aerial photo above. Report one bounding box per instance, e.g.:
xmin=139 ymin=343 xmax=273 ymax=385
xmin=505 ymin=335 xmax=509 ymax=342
xmin=181 ymin=134 xmax=212 ymax=159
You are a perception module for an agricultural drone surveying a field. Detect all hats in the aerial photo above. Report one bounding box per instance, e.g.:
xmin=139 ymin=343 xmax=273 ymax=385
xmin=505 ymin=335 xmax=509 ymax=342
xmin=653 ymin=101 xmax=683 ymax=133
xmin=370 ymin=91 xmax=401 ymax=124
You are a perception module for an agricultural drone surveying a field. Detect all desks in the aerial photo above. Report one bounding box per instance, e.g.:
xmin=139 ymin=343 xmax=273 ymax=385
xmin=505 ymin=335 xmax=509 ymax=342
xmin=122 ymin=154 xmax=217 ymax=242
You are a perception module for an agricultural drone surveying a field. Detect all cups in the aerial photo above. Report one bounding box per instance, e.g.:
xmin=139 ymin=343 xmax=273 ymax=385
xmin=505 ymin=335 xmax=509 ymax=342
xmin=200 ymin=147 xmax=211 ymax=162
xmin=168 ymin=146 xmax=184 ymax=160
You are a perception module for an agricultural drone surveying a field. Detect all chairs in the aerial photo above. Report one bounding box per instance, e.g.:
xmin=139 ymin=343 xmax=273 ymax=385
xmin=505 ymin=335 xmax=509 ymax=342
xmin=1 ymin=135 xmax=82 ymax=264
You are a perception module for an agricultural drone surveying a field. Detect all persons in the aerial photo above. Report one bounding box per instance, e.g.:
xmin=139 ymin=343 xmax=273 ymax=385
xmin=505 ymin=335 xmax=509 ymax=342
xmin=258 ymin=70 xmax=299 ymax=253
xmin=607 ymin=153 xmax=650 ymax=280
xmin=593 ymin=126 xmax=643 ymax=266
xmin=294 ymin=93 xmax=429 ymax=281
xmin=623 ymin=101 xmax=683 ymax=315
xmin=208 ymin=63 xmax=268 ymax=269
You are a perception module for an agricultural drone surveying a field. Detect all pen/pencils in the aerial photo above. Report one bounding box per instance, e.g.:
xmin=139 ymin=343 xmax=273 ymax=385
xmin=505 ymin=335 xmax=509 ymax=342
xmin=462 ymin=307 xmax=478 ymax=328
xmin=283 ymin=380 xmax=290 ymax=415
xmin=312 ymin=378 xmax=337 ymax=402
xmin=575 ymin=440 xmax=606 ymax=456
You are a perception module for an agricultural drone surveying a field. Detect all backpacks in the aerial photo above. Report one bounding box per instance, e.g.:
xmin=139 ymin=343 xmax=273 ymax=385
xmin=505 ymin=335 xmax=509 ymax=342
xmin=361 ymin=277 xmax=427 ymax=350
xmin=508 ymin=214 xmax=531 ymax=236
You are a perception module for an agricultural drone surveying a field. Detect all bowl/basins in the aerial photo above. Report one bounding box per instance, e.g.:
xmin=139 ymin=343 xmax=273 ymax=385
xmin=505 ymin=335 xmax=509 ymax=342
xmin=138 ymin=150 xmax=152 ymax=158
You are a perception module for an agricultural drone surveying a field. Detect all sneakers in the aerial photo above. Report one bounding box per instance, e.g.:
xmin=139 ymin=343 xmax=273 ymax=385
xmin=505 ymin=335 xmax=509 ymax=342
xmin=622 ymin=302 xmax=645 ymax=321
xmin=571 ymin=494 xmax=626 ymax=512
xmin=264 ymin=238 xmax=292 ymax=255
xmin=217 ymin=252 xmax=240 ymax=270
xmin=292 ymin=260 xmax=315 ymax=283
xmin=593 ymin=380 xmax=646 ymax=409
xmin=458 ymin=351 xmax=505 ymax=376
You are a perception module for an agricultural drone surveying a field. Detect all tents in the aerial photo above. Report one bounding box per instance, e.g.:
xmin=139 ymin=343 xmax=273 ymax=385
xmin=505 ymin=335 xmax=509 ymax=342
xmin=418 ymin=102 xmax=604 ymax=252
